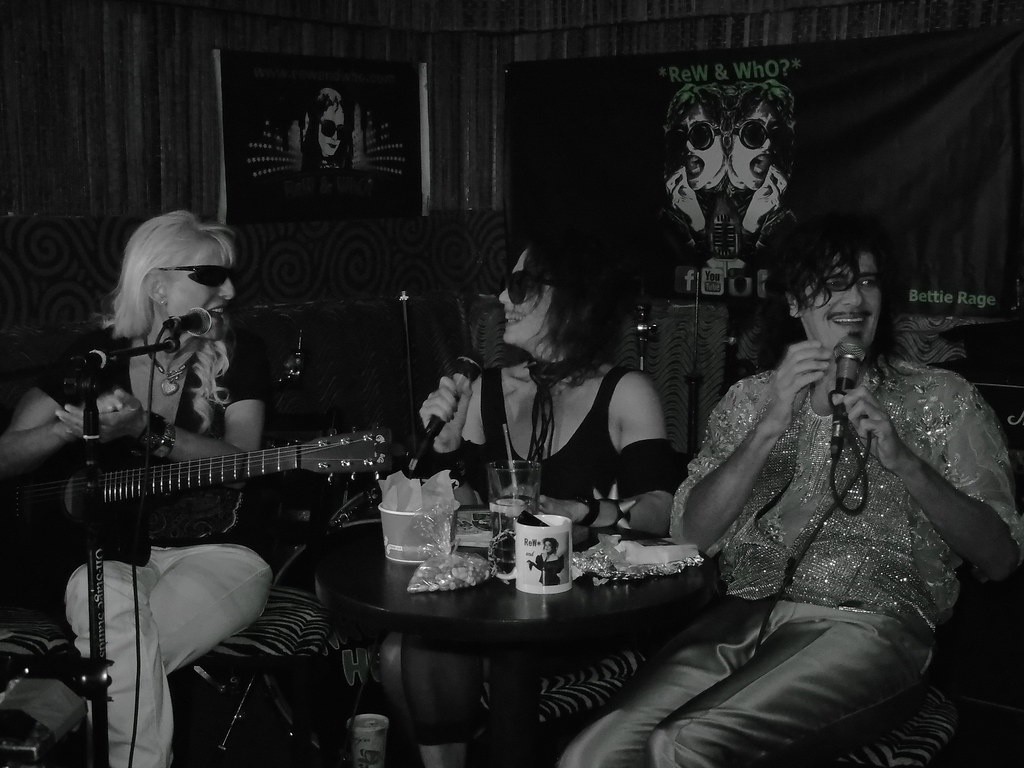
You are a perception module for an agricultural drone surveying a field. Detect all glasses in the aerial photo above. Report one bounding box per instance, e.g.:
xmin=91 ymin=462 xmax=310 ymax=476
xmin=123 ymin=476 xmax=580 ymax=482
xmin=158 ymin=265 xmax=236 ymax=287
xmin=499 ymin=270 xmax=556 ymax=304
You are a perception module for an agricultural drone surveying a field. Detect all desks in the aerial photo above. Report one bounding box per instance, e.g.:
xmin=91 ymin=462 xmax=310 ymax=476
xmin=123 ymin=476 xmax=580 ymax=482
xmin=316 ymin=522 xmax=720 ymax=768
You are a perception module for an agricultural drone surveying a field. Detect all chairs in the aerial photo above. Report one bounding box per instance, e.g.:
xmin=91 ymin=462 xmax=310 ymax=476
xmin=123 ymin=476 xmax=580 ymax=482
xmin=1 ymin=214 xmax=1023 ymax=767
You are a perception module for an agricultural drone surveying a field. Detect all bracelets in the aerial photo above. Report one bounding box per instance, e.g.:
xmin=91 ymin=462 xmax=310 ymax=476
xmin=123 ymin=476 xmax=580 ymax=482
xmin=593 ymin=481 xmax=636 ymax=534
xmin=132 ymin=412 xmax=166 ymax=458
xmin=432 ymin=438 xmax=469 ymax=489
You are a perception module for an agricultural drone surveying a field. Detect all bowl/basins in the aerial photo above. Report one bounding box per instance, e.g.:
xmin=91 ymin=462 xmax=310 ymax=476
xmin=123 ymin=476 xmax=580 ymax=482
xmin=378 ymin=500 xmax=461 ymax=562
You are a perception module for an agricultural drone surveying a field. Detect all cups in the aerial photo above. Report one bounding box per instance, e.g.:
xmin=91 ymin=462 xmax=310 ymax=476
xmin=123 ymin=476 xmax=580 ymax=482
xmin=513 ymin=514 xmax=574 ymax=595
xmin=485 ymin=460 xmax=541 ymax=580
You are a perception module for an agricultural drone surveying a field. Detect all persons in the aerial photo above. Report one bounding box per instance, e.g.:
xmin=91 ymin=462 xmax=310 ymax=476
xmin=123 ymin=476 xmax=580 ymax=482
xmin=554 ymin=211 xmax=1024 ymax=768
xmin=0 ymin=209 xmax=274 ymax=768
xmin=379 ymin=235 xmax=673 ymax=768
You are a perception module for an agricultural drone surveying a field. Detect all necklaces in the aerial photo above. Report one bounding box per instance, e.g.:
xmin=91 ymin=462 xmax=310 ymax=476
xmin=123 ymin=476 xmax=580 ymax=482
xmin=523 ymin=348 xmax=594 ymax=459
xmin=142 ymin=334 xmax=199 ymax=396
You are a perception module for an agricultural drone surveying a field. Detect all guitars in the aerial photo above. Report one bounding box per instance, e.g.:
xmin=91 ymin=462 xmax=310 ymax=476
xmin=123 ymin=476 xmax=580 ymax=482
xmin=0 ymin=421 xmax=393 ymax=567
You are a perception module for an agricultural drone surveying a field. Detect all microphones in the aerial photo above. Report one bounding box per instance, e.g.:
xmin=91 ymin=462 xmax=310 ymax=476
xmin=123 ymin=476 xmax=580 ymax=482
xmin=409 ymin=348 xmax=485 ymax=472
xmin=165 ymin=308 xmax=225 ymax=336
xmin=831 ymin=336 xmax=868 ymax=456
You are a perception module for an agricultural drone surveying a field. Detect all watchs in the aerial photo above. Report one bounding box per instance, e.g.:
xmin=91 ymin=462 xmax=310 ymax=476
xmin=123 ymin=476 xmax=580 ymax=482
xmin=153 ymin=422 xmax=176 ymax=459
xmin=576 ymin=494 xmax=600 ymax=528
